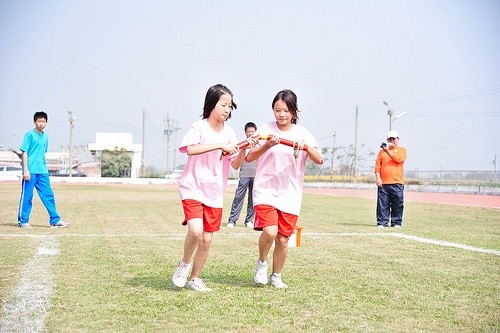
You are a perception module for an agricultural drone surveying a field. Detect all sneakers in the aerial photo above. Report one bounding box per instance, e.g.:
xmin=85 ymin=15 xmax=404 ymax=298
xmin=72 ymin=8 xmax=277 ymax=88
xmin=172 ymin=258 xmax=193 ymax=288
xmin=254 ymin=257 xmax=268 ymax=285
xmin=270 ymin=274 xmax=288 ymax=289
xmin=187 ymin=278 xmax=212 ymax=293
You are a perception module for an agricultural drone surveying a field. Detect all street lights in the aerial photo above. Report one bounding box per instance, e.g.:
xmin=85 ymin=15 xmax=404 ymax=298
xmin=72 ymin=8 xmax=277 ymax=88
xmin=382 ymin=99 xmax=408 ymax=131
xmin=67 ymin=110 xmax=76 ymax=177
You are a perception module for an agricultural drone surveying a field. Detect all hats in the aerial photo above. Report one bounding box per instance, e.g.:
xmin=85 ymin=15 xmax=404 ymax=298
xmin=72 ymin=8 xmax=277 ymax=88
xmin=387 ymin=131 xmax=399 ymax=139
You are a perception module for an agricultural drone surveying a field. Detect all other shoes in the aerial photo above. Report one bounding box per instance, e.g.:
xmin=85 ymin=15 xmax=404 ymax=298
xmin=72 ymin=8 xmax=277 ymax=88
xmin=21 ymin=223 xmax=31 ymax=229
xmin=50 ymin=220 xmax=68 ymax=228
xmin=246 ymin=221 xmax=253 ymax=228
xmin=227 ymin=223 xmax=234 ymax=227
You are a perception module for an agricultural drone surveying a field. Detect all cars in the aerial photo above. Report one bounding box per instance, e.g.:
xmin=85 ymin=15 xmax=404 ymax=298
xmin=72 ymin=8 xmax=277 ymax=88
xmin=0 ymin=166 xmax=22 ymax=181
xmin=49 ymin=168 xmax=87 ymax=177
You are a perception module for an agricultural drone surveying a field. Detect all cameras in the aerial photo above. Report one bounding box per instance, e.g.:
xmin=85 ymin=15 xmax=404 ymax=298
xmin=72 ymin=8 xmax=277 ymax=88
xmin=380 ymin=143 xmax=393 ymax=148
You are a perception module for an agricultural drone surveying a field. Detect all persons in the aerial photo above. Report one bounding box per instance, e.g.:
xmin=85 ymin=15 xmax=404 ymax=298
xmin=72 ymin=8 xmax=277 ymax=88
xmin=18 ymin=111 xmax=71 ymax=228
xmin=243 ymin=89 xmax=324 ymax=289
xmin=226 ymin=121 xmax=265 ymax=228
xmin=172 ymin=83 xmax=260 ymax=291
xmin=375 ymin=130 xmax=406 ymax=228
